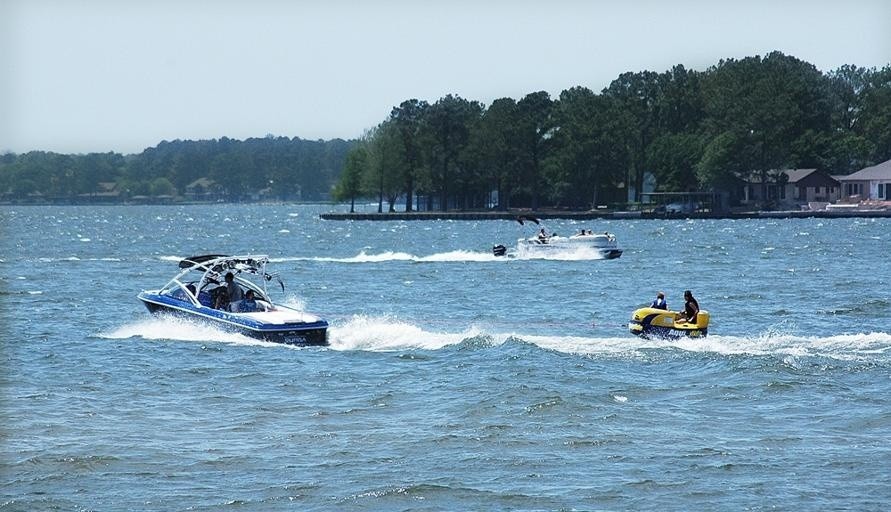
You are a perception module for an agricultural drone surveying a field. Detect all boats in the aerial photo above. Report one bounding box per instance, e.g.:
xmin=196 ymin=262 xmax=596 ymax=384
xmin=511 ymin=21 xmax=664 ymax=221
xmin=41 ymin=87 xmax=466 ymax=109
xmin=508 ymin=215 xmax=623 ymax=259
xmin=137 ymin=253 xmax=328 ymax=344
xmin=629 ymin=308 xmax=709 ymax=341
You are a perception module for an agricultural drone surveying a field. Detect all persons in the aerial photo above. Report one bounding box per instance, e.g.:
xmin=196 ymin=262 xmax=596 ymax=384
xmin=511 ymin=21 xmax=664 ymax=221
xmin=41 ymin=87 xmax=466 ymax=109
xmin=214 ymin=272 xmax=243 ymax=308
xmin=577 ymin=229 xmax=588 ymax=235
xmin=651 ymin=292 xmax=669 ymax=310
xmin=539 ymin=228 xmax=550 ymax=243
xmin=676 ymin=290 xmax=699 ymax=325
xmin=604 ymin=231 xmax=611 ymax=236
xmin=588 ymin=230 xmax=593 ymax=236
xmin=552 ymin=232 xmax=560 ymax=243
xmin=238 ymin=289 xmax=257 ymax=312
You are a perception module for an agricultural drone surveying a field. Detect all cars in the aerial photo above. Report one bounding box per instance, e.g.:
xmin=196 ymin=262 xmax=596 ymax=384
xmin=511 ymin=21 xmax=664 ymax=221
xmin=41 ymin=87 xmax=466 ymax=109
xmin=656 ymin=199 xmax=689 ymax=214
